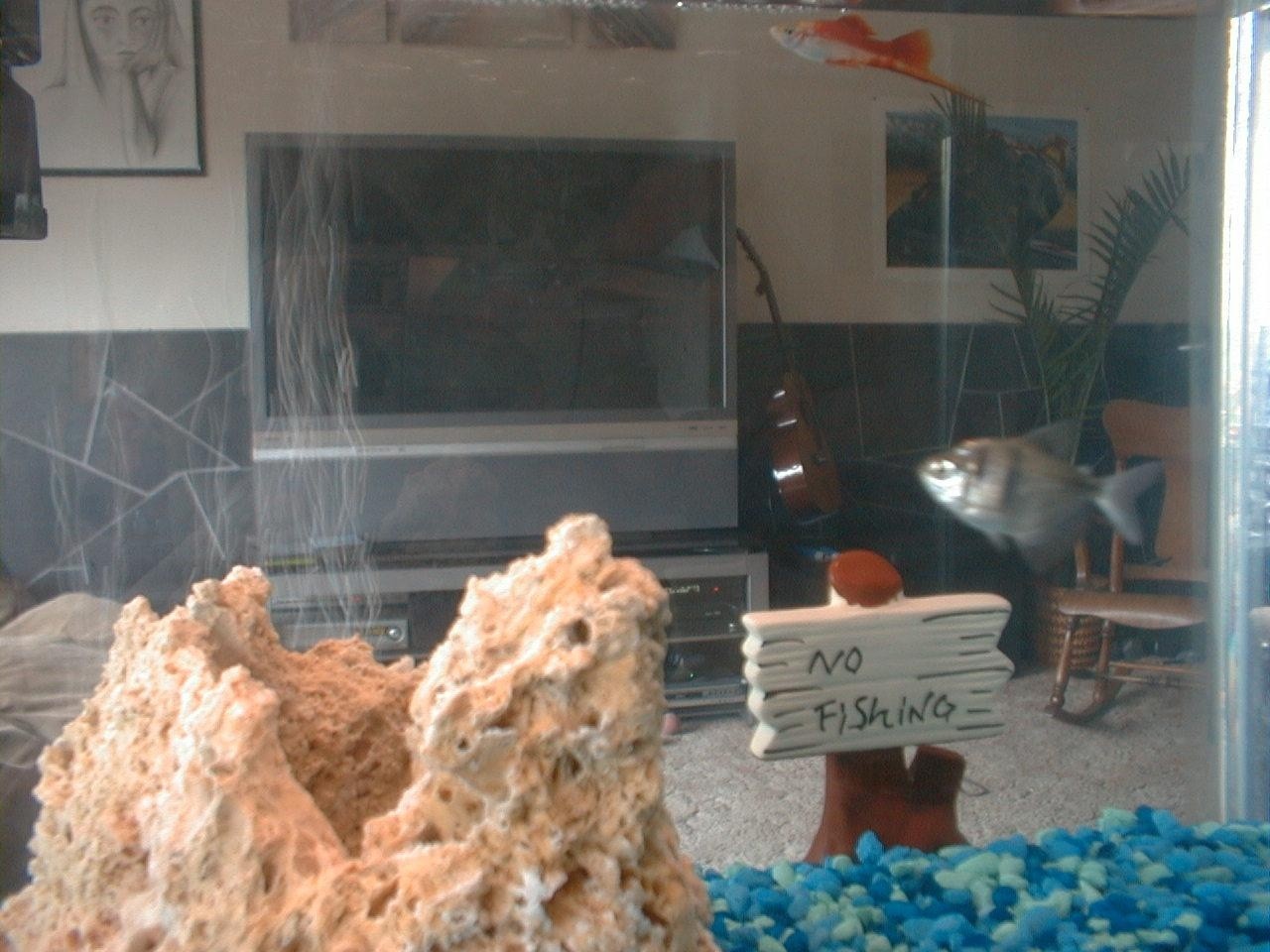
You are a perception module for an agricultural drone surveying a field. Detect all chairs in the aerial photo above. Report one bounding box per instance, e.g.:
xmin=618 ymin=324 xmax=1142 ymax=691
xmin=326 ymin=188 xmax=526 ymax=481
xmin=761 ymin=454 xmax=844 ymax=570
xmin=1044 ymin=399 xmax=1216 ymax=723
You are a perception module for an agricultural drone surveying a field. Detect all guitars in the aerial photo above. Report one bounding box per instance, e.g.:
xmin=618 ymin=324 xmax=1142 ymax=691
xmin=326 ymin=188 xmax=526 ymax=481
xmin=733 ymin=223 xmax=845 ymax=516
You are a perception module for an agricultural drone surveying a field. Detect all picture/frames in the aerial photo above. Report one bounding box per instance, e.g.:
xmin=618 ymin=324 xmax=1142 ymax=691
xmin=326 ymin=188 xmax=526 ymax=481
xmin=12 ymin=0 xmax=205 ymax=176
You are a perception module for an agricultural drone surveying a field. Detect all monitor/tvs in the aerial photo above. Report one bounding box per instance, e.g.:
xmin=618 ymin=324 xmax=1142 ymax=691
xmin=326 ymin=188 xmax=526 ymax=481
xmin=244 ymin=131 xmax=740 ymax=556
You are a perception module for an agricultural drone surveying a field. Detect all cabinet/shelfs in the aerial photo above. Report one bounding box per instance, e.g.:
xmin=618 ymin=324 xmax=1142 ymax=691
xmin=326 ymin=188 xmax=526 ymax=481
xmin=246 ymin=533 xmax=772 ymax=728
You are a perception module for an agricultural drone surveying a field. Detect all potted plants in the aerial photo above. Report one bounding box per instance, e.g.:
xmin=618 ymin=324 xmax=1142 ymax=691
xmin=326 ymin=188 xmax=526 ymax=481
xmin=919 ymin=87 xmax=1214 ymax=672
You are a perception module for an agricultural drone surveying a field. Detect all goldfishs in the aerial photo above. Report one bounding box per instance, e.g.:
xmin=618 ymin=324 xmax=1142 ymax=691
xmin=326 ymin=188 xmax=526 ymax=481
xmin=919 ymin=422 xmax=1161 ymax=576
xmin=769 ymin=15 xmax=956 ymax=92
